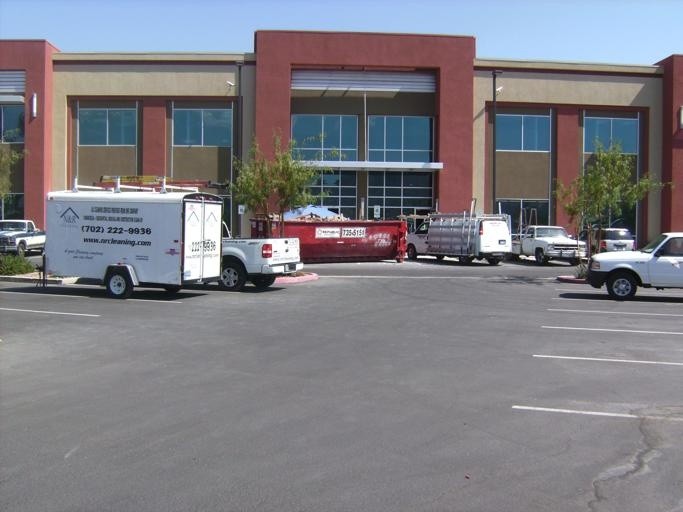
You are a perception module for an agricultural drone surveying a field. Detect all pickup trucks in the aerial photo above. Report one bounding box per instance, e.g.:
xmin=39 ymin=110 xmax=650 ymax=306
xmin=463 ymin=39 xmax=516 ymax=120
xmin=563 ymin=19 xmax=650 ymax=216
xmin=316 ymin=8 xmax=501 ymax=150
xmin=0 ymin=219 xmax=45 ymax=258
xmin=407 ymin=201 xmax=682 ymax=303
xmin=220 ymin=222 xmax=304 ymax=289
